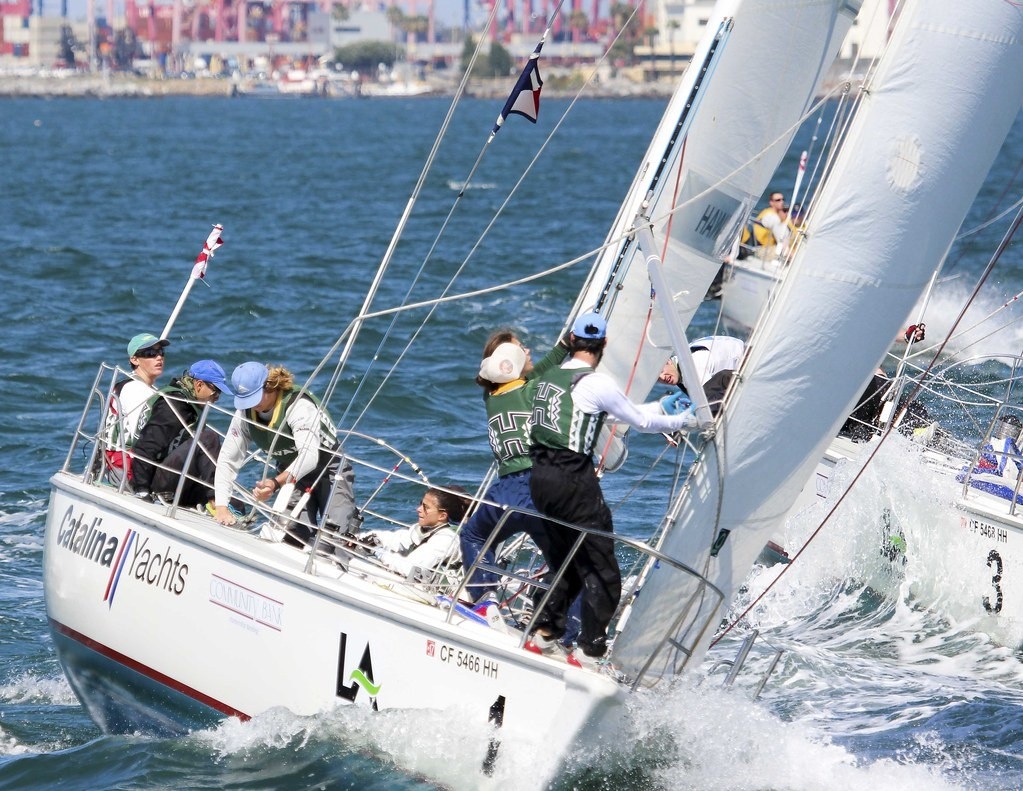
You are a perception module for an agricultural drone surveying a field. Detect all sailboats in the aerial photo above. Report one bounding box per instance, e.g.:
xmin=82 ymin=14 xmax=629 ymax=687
xmin=44 ymin=0 xmax=1023 ymax=791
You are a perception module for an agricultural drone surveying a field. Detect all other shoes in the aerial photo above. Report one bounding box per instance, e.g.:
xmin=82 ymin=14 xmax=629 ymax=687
xmin=568 ymin=647 xmax=607 ymax=673
xmin=525 ymin=634 xmax=567 ymax=661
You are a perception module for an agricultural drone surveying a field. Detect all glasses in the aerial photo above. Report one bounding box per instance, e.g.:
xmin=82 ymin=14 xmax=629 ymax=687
xmin=205 ymin=381 xmax=221 ymax=394
xmin=134 ymin=347 xmax=164 ymax=358
xmin=772 ymin=199 xmax=783 ymax=202
xmin=419 ymin=502 xmax=448 ymax=514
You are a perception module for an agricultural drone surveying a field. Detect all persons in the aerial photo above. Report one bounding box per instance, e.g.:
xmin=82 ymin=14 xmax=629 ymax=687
xmin=126 ymin=359 xmax=254 ymax=528
xmin=754 ymin=192 xmax=808 ymax=258
xmin=658 ymin=335 xmax=747 ymax=417
xmin=349 ymin=485 xmax=478 ymax=588
xmin=529 ymin=314 xmax=698 ymax=663
xmin=212 ymin=361 xmax=357 ymax=568
xmin=955 ymin=414 xmax=1023 ymax=505
xmin=841 ymin=323 xmax=926 ymax=444
xmin=460 ymin=331 xmax=583 ymax=648
xmin=105 ymin=333 xmax=171 ymax=491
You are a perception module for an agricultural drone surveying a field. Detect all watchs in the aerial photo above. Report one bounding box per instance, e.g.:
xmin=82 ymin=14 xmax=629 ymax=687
xmin=271 ymin=478 xmax=280 ymax=493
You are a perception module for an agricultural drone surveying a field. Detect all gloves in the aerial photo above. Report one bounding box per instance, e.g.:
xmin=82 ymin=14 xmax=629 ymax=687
xmin=681 ymin=403 xmax=699 ymax=432
xmin=662 ymin=391 xmax=691 ymax=416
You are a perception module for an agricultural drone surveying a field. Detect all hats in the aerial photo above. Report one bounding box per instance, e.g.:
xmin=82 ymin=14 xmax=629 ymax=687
xmin=127 ymin=333 xmax=171 ymax=358
xmin=189 ymin=359 xmax=235 ymax=396
xmin=231 ymin=361 xmax=269 ymax=410
xmin=480 ymin=343 xmax=526 ymax=384
xmin=791 ymin=204 xmax=804 ymax=216
xmin=571 ymin=313 xmax=606 ymax=338
